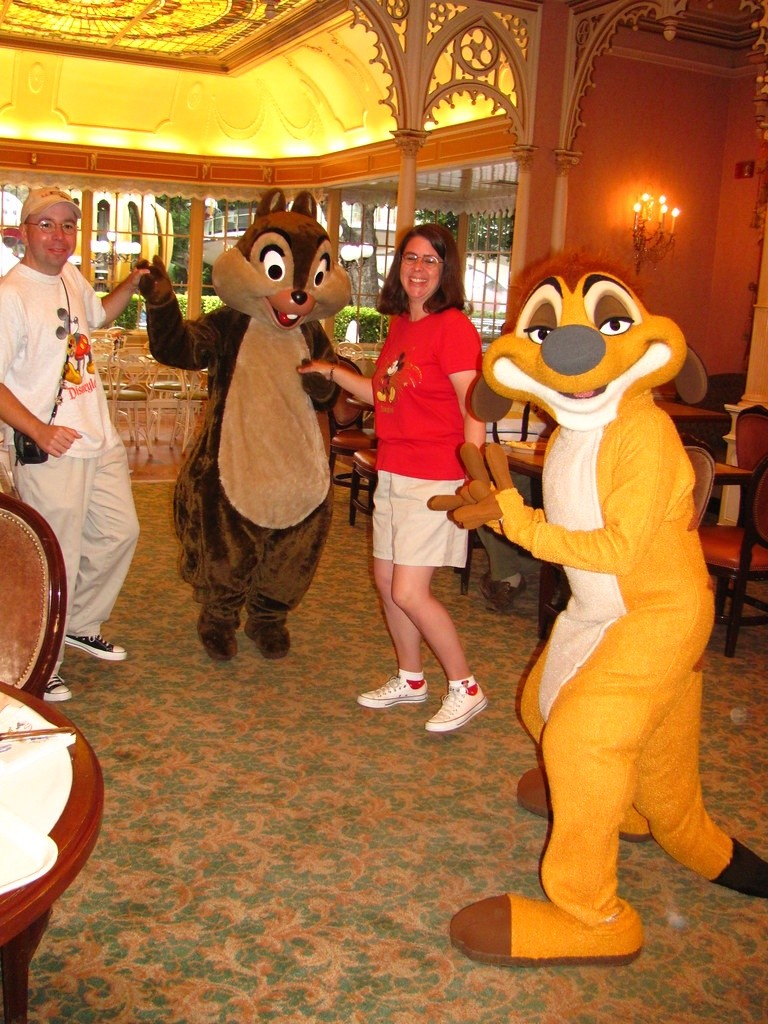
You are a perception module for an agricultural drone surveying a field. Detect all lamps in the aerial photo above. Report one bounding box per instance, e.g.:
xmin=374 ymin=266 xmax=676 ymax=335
xmin=632 ymin=192 xmax=680 ymax=276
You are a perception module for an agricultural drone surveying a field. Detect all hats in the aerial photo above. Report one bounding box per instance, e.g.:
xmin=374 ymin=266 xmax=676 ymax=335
xmin=21 ymin=187 xmax=82 ymax=223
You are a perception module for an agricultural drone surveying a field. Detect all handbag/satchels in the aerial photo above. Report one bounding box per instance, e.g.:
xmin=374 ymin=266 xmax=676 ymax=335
xmin=14 ymin=430 xmax=48 ymax=466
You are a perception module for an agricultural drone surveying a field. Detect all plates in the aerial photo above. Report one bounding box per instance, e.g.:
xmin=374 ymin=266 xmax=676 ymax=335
xmin=1 ymin=816 xmax=58 ymax=893
xmin=505 ymin=441 xmax=546 ymax=455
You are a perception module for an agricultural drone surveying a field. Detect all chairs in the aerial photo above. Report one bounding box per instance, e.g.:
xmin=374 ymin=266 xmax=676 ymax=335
xmin=88 ymin=326 xmax=768 ymax=660
xmin=0 ymin=493 xmax=67 ymax=700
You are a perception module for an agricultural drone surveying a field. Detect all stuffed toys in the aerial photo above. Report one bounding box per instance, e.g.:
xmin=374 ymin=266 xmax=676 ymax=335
xmin=133 ymin=189 xmax=350 ymax=662
xmin=429 ymin=252 xmax=766 ymax=965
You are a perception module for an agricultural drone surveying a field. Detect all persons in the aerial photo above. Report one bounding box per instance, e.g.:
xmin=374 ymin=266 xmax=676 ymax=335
xmin=0 ymin=185 xmax=150 ymax=703
xmin=296 ymin=223 xmax=490 ymax=735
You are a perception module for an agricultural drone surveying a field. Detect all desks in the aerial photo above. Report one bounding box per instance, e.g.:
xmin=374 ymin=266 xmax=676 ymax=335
xmin=460 ymin=441 xmax=752 ymax=638
xmin=0 ymin=682 xmax=105 ymax=1024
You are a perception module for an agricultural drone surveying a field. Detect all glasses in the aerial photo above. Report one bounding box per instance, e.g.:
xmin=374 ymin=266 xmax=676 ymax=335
xmin=401 ymin=252 xmax=444 ymax=269
xmin=26 ymin=220 xmax=80 ymax=235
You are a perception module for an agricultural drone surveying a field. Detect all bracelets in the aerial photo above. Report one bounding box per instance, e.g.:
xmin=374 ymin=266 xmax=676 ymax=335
xmin=329 ymin=364 xmax=335 ymax=379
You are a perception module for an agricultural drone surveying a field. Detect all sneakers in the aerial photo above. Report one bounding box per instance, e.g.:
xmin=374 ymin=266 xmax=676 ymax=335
xmin=425 ymin=683 xmax=488 ymax=732
xmin=356 ymin=675 xmax=428 ymax=708
xmin=65 ymin=634 xmax=127 ymax=660
xmin=43 ymin=676 xmax=72 ymax=701
